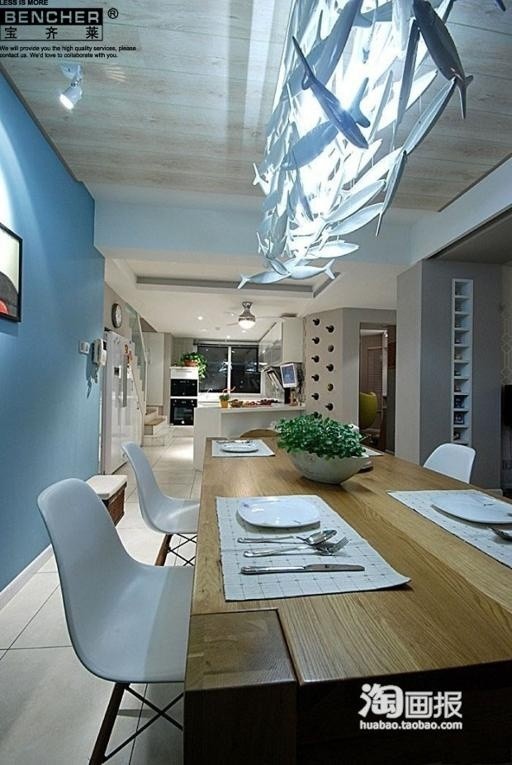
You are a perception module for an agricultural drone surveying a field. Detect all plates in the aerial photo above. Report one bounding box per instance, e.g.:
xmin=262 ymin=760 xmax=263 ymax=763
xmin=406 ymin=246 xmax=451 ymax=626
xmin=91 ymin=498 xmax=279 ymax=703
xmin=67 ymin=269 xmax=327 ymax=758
xmin=430 ymin=501 xmax=512 ymax=524
xmin=236 ymin=497 xmax=322 ymax=528
xmin=223 ymin=442 xmax=258 ymax=452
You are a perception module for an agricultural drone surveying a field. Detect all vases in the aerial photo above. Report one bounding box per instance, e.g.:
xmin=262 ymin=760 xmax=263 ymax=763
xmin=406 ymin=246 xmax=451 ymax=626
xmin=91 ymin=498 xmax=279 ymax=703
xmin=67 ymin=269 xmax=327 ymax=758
xmin=219 ymin=394 xmax=231 ymax=408
xmin=180 ymin=352 xmax=208 ymax=379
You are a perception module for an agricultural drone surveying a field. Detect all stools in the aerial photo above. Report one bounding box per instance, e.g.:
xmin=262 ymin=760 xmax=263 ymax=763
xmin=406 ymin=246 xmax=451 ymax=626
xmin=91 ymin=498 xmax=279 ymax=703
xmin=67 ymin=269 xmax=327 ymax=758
xmin=84 ymin=474 xmax=127 ymax=527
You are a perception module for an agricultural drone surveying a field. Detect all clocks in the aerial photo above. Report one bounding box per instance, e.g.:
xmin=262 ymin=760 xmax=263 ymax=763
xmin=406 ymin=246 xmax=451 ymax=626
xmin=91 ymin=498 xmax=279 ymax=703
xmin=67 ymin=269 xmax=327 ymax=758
xmin=112 ymin=303 xmax=123 ymax=328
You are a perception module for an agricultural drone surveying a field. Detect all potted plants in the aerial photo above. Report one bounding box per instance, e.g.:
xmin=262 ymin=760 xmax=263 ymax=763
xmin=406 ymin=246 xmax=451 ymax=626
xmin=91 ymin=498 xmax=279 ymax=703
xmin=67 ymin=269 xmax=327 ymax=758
xmin=274 ymin=411 xmax=369 ymax=485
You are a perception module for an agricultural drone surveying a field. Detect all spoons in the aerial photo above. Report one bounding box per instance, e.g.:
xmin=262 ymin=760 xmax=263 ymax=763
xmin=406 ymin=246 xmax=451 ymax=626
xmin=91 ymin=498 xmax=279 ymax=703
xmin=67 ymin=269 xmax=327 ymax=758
xmin=490 ymin=526 xmax=512 ymax=543
xmin=238 ymin=530 xmax=338 ymax=546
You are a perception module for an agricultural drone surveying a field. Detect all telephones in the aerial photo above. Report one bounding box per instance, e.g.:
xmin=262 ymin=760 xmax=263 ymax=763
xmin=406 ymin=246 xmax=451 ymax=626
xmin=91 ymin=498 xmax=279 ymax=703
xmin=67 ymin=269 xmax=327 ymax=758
xmin=93 ymin=338 xmax=108 ymax=384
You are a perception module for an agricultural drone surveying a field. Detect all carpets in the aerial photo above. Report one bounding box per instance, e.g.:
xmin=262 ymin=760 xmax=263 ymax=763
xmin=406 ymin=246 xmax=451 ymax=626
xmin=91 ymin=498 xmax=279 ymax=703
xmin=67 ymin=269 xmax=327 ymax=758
xmin=172 ymin=426 xmax=194 ymax=438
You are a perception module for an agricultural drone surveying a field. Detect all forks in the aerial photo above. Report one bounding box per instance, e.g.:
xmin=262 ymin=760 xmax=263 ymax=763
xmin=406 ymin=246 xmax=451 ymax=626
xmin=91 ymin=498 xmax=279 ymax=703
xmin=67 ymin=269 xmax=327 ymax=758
xmin=243 ymin=537 xmax=350 ymax=557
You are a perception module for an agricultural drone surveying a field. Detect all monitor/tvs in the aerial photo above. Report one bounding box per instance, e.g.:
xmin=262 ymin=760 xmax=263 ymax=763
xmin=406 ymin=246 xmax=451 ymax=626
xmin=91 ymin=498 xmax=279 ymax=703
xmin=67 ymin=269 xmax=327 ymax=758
xmin=280 ymin=363 xmax=299 ymax=388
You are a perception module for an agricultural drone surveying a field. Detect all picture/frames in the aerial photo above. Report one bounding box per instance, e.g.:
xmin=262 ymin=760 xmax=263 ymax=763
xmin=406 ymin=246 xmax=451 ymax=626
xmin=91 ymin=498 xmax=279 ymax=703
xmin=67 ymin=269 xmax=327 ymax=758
xmin=1 ymin=223 xmax=24 ymax=323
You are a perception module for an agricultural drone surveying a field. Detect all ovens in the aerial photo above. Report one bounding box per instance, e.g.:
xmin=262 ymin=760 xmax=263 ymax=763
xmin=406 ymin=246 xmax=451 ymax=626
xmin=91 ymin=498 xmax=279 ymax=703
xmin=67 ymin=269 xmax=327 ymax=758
xmin=169 ymin=379 xmax=198 ymax=437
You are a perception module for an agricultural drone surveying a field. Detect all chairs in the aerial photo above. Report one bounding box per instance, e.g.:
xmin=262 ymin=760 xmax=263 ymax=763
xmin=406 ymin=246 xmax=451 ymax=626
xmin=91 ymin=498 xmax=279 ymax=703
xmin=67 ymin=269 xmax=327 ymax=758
xmin=123 ymin=440 xmax=200 ymax=566
xmin=422 ymin=442 xmax=475 ymax=485
xmin=37 ymin=478 xmax=195 ymax=764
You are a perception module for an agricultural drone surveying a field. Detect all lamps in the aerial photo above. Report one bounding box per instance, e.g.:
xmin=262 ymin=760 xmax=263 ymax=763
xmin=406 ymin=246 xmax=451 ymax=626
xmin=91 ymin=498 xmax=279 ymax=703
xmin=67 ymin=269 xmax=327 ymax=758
xmin=238 ymin=301 xmax=256 ymax=330
xmin=239 ymin=0 xmax=474 ymax=296
xmin=58 ymin=63 xmax=84 ymax=110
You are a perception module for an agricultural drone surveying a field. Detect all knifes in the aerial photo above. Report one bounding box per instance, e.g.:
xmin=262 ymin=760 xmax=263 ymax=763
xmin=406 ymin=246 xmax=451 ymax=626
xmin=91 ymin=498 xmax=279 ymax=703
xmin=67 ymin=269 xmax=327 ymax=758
xmin=240 ymin=563 xmax=366 ymax=575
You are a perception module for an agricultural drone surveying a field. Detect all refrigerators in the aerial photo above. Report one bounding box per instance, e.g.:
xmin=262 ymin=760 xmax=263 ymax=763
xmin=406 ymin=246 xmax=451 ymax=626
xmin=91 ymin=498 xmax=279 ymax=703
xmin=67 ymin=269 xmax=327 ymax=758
xmin=103 ymin=326 xmax=136 ymax=475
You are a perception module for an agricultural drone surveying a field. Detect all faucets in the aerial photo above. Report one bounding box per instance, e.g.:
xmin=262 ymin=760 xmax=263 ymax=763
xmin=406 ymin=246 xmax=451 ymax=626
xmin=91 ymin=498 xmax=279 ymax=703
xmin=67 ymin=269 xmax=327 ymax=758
xmin=206 ymin=388 xmax=213 ymax=399
